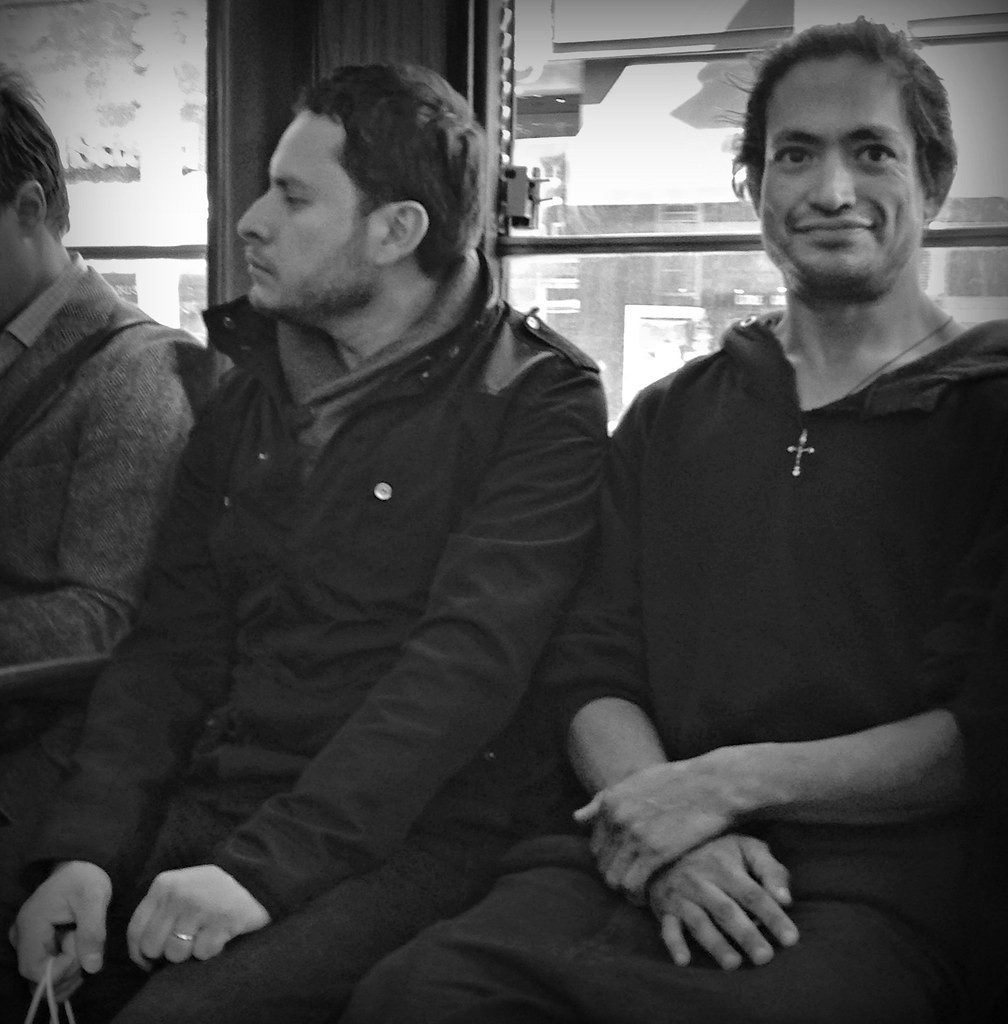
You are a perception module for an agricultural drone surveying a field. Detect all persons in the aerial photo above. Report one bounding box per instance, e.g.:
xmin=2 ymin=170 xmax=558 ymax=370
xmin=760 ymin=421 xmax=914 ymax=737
xmin=0 ymin=64 xmax=211 ymax=1023
xmin=0 ymin=64 xmax=607 ymax=1023
xmin=339 ymin=15 xmax=1008 ymax=1024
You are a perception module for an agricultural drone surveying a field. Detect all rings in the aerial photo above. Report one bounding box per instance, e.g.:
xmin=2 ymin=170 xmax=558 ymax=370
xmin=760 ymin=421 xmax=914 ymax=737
xmin=172 ymin=929 xmax=195 ymax=941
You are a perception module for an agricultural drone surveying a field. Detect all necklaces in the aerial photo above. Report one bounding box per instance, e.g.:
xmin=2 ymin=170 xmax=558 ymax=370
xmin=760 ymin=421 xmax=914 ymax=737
xmin=787 ymin=319 xmax=952 ymax=478
